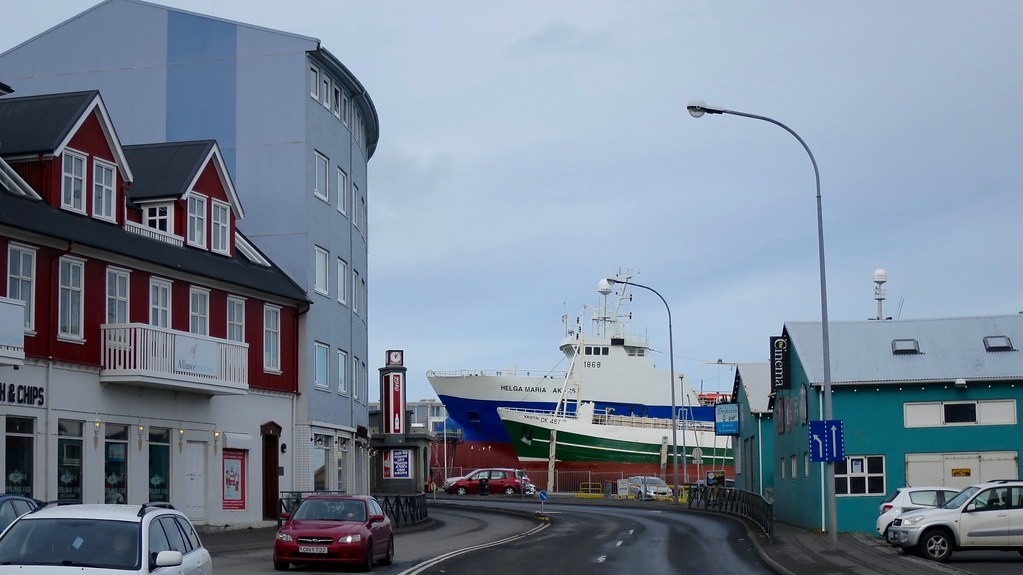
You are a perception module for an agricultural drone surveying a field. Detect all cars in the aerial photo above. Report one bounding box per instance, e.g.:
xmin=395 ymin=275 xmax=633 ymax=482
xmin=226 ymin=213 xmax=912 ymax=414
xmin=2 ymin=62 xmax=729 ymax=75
xmin=273 ymin=494 xmax=395 ymax=572
xmin=444 ymin=469 xmax=536 ymax=495
xmin=0 ymin=499 xmax=213 ymax=575
xmin=446 ymin=468 xmax=526 ymax=495
xmin=875 ymin=487 xmax=988 ymax=541
xmin=0 ymin=493 xmax=38 ymax=535
xmin=627 ymin=476 xmax=673 ymax=500
xmin=694 ymin=479 xmax=735 ymax=498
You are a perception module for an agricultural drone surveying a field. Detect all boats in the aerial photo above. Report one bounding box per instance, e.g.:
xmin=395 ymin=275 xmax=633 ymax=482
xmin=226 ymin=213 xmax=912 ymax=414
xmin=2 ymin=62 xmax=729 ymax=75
xmin=498 ymin=407 xmax=735 ymax=487
xmin=426 ymin=280 xmax=717 ymax=487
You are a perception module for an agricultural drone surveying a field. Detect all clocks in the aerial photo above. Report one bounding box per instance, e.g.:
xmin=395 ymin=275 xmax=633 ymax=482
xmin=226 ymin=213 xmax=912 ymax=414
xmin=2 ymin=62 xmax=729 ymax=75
xmin=390 ymin=351 xmax=401 ymax=362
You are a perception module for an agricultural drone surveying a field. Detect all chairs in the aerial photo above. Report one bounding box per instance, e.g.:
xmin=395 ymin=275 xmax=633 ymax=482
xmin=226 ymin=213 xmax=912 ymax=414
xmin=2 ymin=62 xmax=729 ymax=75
xmin=988 ymin=491 xmax=1007 ymax=510
xmin=314 ymin=503 xmax=328 ymax=519
xmin=42 ymin=529 xmax=76 ymax=561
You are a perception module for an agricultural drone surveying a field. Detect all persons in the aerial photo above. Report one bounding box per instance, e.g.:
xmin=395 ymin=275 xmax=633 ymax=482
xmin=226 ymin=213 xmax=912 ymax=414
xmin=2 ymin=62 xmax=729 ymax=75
xmin=109 ymin=526 xmax=137 ymax=566
xmin=346 ymin=504 xmax=362 ymax=522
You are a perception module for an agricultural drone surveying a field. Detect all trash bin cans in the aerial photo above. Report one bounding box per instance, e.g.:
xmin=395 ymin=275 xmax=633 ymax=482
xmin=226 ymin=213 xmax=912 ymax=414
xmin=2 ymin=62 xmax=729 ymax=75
xmin=479 ymin=478 xmax=489 ymax=495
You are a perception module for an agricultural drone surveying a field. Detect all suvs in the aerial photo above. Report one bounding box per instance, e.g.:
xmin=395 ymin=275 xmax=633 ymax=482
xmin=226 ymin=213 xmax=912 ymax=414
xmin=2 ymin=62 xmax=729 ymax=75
xmin=888 ymin=479 xmax=1023 ymax=562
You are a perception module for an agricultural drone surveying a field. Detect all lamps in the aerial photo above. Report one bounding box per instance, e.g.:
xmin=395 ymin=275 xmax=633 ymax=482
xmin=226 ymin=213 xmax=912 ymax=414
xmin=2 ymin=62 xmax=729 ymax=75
xmin=137 ymin=421 xmax=145 ymax=452
xmin=177 ymin=426 xmax=185 ymax=455
xmin=93 ymin=418 xmax=101 ymax=450
xmin=213 ymin=430 xmax=221 ymax=456
xmin=315 ymin=437 xmax=378 ymax=455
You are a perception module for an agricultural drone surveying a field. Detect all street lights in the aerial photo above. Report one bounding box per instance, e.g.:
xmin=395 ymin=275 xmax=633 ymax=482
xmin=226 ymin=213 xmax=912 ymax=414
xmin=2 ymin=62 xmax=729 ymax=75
xmin=606 ymin=275 xmax=677 ymax=510
xmin=687 ymin=100 xmax=839 ymax=554
